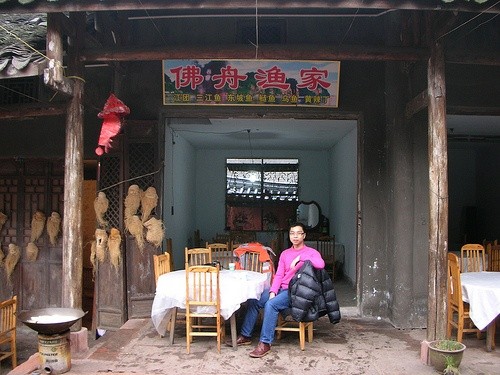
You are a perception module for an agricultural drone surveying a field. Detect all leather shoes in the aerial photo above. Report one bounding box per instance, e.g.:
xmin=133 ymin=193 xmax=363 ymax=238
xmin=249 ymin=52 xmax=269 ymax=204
xmin=250 ymin=342 xmax=271 ymax=357
xmin=225 ymin=335 xmax=252 ymax=346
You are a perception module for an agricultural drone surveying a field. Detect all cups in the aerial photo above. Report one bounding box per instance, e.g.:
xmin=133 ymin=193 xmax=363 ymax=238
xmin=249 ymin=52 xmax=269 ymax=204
xmin=229 ymin=262 xmax=235 ymax=271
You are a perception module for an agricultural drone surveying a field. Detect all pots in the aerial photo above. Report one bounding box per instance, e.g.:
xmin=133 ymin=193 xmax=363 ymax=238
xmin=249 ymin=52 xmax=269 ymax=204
xmin=13 ymin=307 xmax=89 ymax=335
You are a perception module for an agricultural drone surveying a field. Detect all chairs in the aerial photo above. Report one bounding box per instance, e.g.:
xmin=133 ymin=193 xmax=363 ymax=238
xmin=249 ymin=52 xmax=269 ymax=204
xmin=153 ymin=228 xmax=336 ymax=354
xmin=446 ymin=242 xmax=500 ymax=349
xmin=0 ymin=296 xmax=18 ymax=370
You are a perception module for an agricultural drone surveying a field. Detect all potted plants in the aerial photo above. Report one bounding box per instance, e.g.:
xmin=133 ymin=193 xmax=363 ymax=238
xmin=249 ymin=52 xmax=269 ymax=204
xmin=427 ymin=338 xmax=466 ymax=375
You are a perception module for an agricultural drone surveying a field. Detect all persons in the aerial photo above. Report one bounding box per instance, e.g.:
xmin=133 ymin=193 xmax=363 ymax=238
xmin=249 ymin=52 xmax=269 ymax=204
xmin=225 ymin=222 xmax=325 ymax=357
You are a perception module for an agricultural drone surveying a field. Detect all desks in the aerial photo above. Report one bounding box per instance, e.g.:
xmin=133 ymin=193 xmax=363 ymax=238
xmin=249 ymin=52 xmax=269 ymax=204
xmin=306 ymin=239 xmax=344 ymax=264
xmin=450 ymin=271 xmax=500 ymax=352
xmin=151 ymin=267 xmax=271 ymax=350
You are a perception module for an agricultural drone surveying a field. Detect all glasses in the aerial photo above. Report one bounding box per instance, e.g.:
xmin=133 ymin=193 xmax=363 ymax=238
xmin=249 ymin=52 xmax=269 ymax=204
xmin=289 ymin=231 xmax=305 ymax=237
